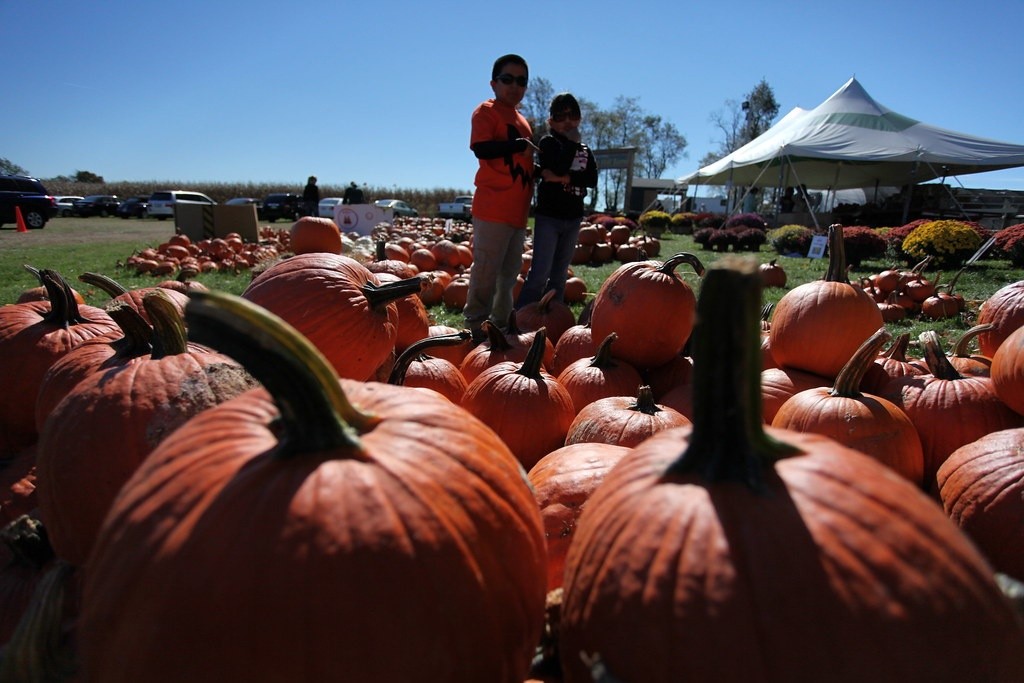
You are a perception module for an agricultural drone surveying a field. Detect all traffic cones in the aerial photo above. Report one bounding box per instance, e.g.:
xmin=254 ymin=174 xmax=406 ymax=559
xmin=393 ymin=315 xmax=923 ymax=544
xmin=16 ymin=206 xmax=28 ymax=233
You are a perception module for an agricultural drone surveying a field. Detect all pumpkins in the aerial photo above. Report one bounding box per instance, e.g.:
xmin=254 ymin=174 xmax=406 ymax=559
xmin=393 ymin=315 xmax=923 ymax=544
xmin=0 ymin=216 xmax=1024 ymax=683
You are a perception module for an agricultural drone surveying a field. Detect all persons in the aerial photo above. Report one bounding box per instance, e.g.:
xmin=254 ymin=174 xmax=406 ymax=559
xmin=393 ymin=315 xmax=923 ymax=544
xmin=342 ymin=182 xmax=364 ymax=204
xmin=463 ymin=54 xmax=541 ymax=342
xmin=515 ymin=92 xmax=598 ymax=311
xmin=743 ymin=187 xmax=758 ymax=212
xmin=304 ymin=177 xmax=320 ymax=216
xmin=780 ymin=184 xmax=813 ymax=213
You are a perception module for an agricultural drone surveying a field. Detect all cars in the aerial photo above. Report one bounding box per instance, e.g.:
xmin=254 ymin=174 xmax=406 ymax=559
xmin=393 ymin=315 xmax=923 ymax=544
xmin=116 ymin=196 xmax=149 ymax=219
xmin=227 ymin=198 xmax=266 ymax=221
xmin=317 ymin=198 xmax=349 ymax=218
xmin=53 ymin=196 xmax=85 ymax=217
xmin=262 ymin=194 xmax=306 ymax=222
xmin=374 ymin=199 xmax=419 ymax=218
xmin=73 ymin=195 xmax=120 ymax=218
xmin=0 ymin=174 xmax=58 ymax=229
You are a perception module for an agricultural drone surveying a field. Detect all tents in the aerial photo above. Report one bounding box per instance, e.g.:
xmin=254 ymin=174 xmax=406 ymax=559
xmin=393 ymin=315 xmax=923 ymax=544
xmin=678 ymin=76 xmax=1024 ymax=226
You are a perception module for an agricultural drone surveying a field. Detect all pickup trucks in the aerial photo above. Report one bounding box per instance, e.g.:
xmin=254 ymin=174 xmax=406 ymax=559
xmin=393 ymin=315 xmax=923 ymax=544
xmin=437 ymin=196 xmax=474 ymax=221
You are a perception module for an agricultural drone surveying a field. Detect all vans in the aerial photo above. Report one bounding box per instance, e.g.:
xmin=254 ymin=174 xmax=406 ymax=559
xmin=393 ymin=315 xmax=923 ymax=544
xmin=146 ymin=191 xmax=217 ymax=220
xmin=679 ymin=194 xmax=727 ymax=214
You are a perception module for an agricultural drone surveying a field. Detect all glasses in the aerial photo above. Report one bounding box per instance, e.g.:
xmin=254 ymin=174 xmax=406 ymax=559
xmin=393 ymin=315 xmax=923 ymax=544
xmin=492 ymin=73 xmax=527 ymax=88
xmin=550 ymin=111 xmax=581 ymax=123
